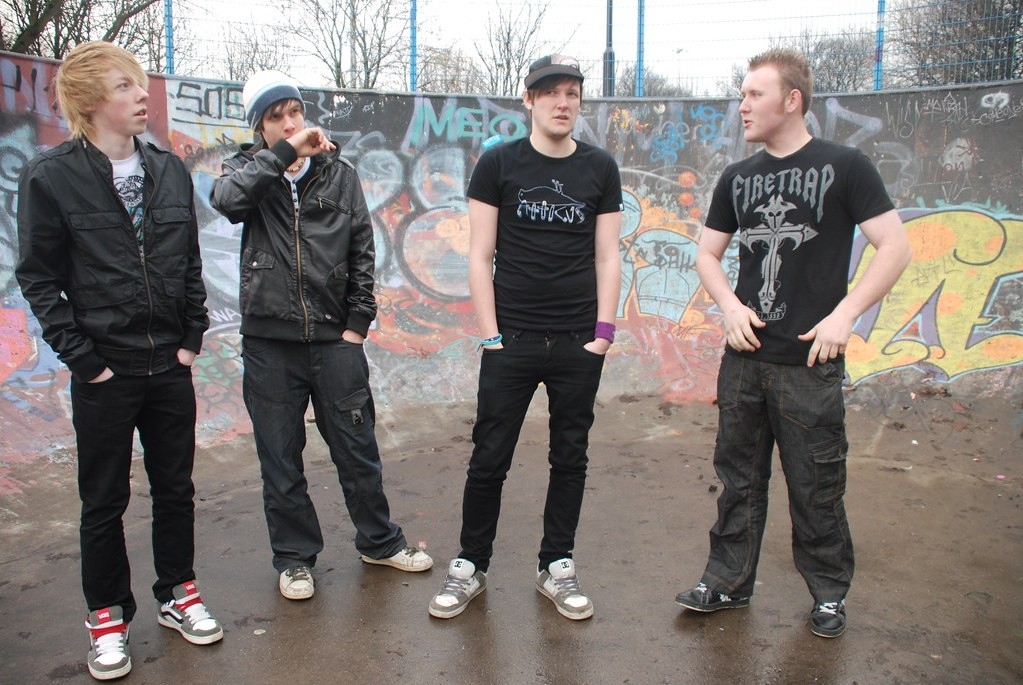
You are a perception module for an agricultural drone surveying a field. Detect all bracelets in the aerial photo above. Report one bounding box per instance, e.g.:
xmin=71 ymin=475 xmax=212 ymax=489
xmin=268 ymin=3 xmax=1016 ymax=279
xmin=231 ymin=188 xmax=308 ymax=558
xmin=477 ymin=334 xmax=502 ymax=352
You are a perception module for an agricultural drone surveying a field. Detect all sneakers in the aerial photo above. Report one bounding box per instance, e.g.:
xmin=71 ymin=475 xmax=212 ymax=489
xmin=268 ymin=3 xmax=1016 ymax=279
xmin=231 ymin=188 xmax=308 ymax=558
xmin=278 ymin=566 xmax=315 ymax=599
xmin=676 ymin=582 xmax=750 ymax=612
xmin=362 ymin=544 xmax=434 ymax=571
xmin=535 ymin=558 xmax=594 ymax=620
xmin=156 ymin=579 xmax=224 ymax=645
xmin=810 ymin=598 xmax=846 ymax=638
xmin=84 ymin=605 xmax=132 ymax=680
xmin=428 ymin=556 xmax=490 ymax=619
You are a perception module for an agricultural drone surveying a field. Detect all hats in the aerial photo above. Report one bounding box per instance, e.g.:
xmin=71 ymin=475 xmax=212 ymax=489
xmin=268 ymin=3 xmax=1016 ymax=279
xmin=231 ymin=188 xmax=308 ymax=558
xmin=523 ymin=53 xmax=584 ymax=89
xmin=242 ymin=70 xmax=306 ymax=132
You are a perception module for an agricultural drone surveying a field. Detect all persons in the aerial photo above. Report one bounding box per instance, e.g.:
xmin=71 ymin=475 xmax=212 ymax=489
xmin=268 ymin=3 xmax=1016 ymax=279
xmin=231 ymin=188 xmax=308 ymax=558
xmin=16 ymin=41 xmax=225 ymax=681
xmin=421 ymin=52 xmax=625 ymax=622
xmin=206 ymin=67 xmax=431 ymax=599
xmin=672 ymin=47 xmax=912 ymax=638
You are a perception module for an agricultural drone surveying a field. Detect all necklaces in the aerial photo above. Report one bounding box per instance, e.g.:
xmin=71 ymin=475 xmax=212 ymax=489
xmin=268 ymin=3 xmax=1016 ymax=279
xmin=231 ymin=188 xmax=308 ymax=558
xmin=282 ymin=156 xmax=308 ymax=172
